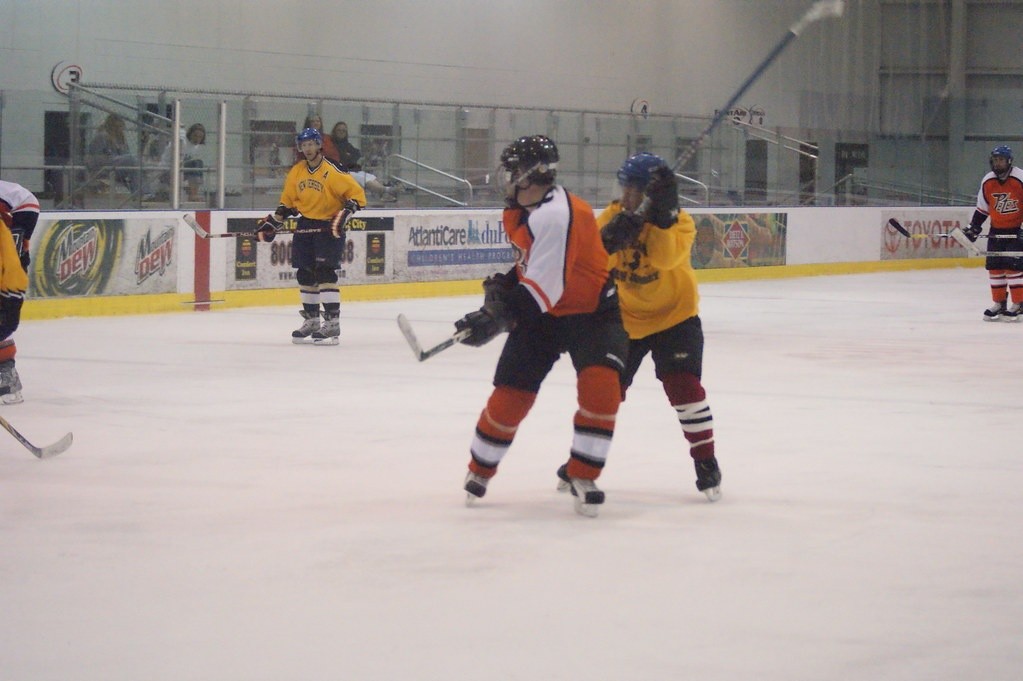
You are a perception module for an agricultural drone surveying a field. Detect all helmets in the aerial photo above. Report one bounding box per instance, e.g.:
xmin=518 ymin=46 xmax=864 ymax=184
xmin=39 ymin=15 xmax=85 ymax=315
xmin=616 ymin=152 xmax=667 ymax=192
xmin=296 ymin=128 xmax=322 ymax=144
xmin=497 ymin=134 xmax=559 ymax=186
xmin=989 ymin=145 xmax=1014 ymax=174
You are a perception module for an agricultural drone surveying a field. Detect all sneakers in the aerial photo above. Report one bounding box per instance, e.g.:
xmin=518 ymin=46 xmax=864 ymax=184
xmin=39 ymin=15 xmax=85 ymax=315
xmin=464 ymin=468 xmax=489 ymax=504
xmin=312 ymin=310 xmax=341 ymax=345
xmin=984 ymin=292 xmax=1009 ymax=321
xmin=571 ymin=476 xmax=606 ymax=516
xmin=555 ymin=462 xmax=571 ymax=491
xmin=0 ymin=357 xmax=23 ymax=405
xmin=694 ymin=454 xmax=723 ymax=503
xmin=1003 ymin=302 xmax=1023 ymax=321
xmin=291 ymin=310 xmax=322 ymax=343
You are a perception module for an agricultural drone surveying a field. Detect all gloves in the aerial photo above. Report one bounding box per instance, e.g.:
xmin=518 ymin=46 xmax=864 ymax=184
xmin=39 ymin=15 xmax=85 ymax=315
xmin=480 ymin=271 xmax=516 ymax=332
xmin=1017 ymin=222 xmax=1023 ymax=241
xmin=962 ymin=225 xmax=983 ymax=242
xmin=12 ymin=236 xmax=31 ymax=272
xmin=453 ymin=299 xmax=510 ymax=345
xmin=254 ymin=213 xmax=285 ymax=242
xmin=600 ymin=212 xmax=644 ymax=254
xmin=646 ymin=165 xmax=679 ymax=227
xmin=331 ymin=208 xmax=353 ymax=239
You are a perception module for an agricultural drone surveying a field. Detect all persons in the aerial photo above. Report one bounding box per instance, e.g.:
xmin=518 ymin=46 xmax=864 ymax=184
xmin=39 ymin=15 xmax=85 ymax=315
xmin=463 ymin=134 xmax=629 ymax=502
xmin=557 ymin=153 xmax=722 ymax=489
xmin=254 ymin=127 xmax=367 ymax=338
xmin=158 ymin=123 xmax=206 ymax=202
xmin=295 ymin=112 xmax=341 ymax=166
xmin=963 ymin=145 xmax=1023 ymax=317
xmin=331 ymin=121 xmax=401 ymax=202
xmin=85 ymin=112 xmax=155 ymax=209
xmin=0 ymin=181 xmax=40 ymax=397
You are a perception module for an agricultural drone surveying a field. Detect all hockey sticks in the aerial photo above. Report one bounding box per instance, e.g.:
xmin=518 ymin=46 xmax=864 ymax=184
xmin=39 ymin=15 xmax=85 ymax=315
xmin=950 ymin=226 xmax=1023 ymax=258
xmin=396 ymin=312 xmax=472 ymax=362
xmin=0 ymin=416 xmax=76 ymax=460
xmin=634 ymin=1 xmax=843 ymax=218
xmin=888 ymin=218 xmax=1017 ymax=240
xmin=182 ymin=213 xmax=331 ymax=239
